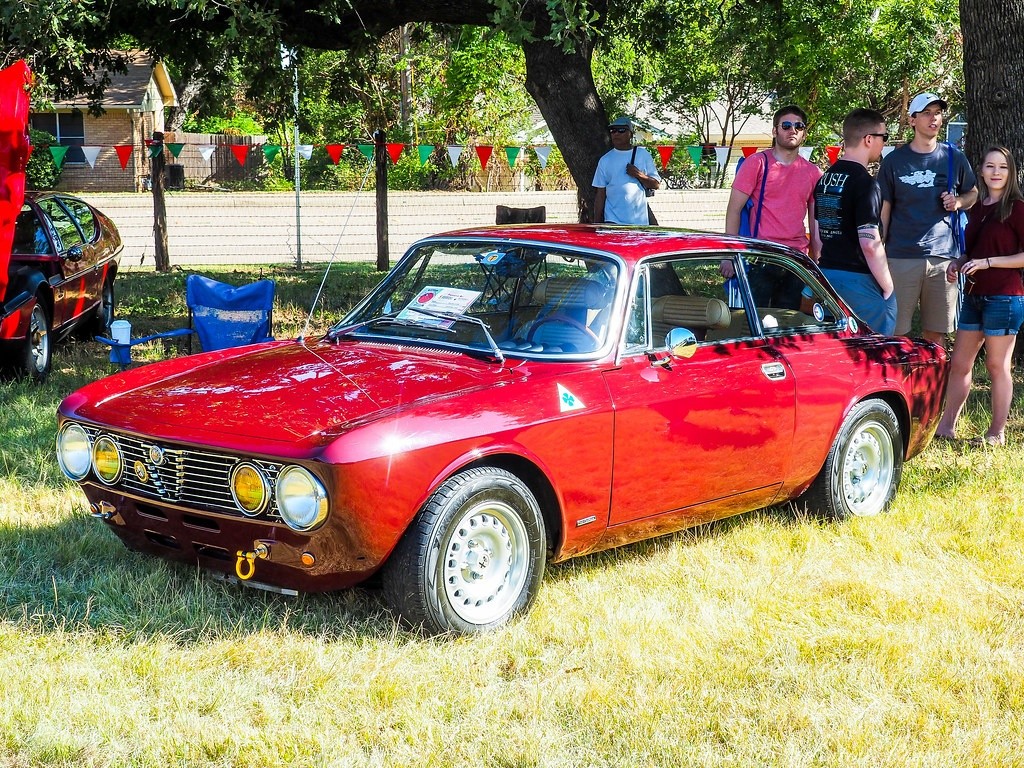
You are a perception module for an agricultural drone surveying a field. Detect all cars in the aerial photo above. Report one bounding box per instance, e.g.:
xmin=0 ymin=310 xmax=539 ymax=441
xmin=0 ymin=189 xmax=125 ymax=386
xmin=57 ymin=219 xmax=953 ymax=647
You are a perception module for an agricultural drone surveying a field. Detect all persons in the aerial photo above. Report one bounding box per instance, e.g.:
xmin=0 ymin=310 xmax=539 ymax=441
xmin=591 ymin=117 xmax=660 ymax=225
xmin=935 ymin=146 xmax=1024 ymax=450
xmin=720 ymin=105 xmax=823 ymax=310
xmin=813 ymin=108 xmax=897 ymax=337
xmin=876 ymin=92 xmax=978 ymax=347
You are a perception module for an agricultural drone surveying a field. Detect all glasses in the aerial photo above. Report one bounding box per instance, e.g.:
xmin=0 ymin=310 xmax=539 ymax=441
xmin=863 ymin=133 xmax=889 ymax=142
xmin=777 ymin=121 xmax=804 ymax=131
xmin=609 ymin=127 xmax=630 ymax=133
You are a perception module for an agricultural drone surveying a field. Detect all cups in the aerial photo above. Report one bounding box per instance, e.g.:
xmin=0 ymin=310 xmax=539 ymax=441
xmin=110 ymin=321 xmax=131 ymax=345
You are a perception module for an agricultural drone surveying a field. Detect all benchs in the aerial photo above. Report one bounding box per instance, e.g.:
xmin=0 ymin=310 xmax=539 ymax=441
xmin=626 ymin=298 xmax=816 ymax=342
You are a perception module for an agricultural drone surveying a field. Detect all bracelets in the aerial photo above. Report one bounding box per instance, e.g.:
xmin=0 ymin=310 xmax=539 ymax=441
xmin=987 ymin=258 xmax=991 ymax=267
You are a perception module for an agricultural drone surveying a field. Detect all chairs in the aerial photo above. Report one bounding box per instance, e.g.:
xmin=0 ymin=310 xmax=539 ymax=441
xmin=96 ymin=273 xmax=274 ymax=372
xmin=513 ymin=277 xmax=611 ymax=352
xmin=473 ymin=205 xmax=548 ymax=310
xmin=14 ymin=210 xmax=52 ymax=254
xmin=652 ymin=294 xmax=732 ymax=347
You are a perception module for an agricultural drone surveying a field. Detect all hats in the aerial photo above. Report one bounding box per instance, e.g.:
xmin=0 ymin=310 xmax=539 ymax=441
xmin=907 ymin=92 xmax=948 ymax=118
xmin=608 ymin=117 xmax=634 ymax=133
